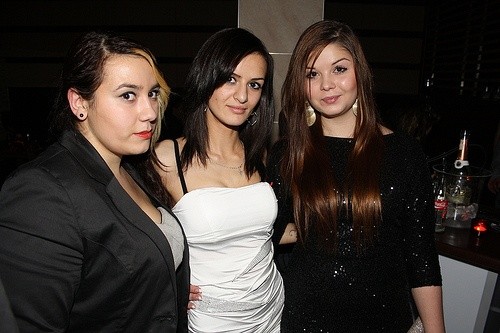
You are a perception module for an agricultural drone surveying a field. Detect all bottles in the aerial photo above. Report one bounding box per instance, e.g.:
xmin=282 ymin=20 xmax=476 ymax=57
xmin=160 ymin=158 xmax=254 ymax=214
xmin=434 ymin=154 xmax=449 ymax=232
xmin=446 ymin=127 xmax=473 ymax=218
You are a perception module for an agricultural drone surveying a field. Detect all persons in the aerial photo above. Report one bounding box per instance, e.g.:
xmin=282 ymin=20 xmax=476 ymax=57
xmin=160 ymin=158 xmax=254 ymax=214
xmin=262 ymin=20 xmax=447 ymax=333
xmin=150 ymin=27 xmax=285 ymax=333
xmin=0 ymin=32 xmax=190 ymax=333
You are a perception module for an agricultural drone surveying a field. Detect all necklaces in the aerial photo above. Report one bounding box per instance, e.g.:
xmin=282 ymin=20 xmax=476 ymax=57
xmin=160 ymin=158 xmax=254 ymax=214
xmin=206 ymin=139 xmax=246 ymax=177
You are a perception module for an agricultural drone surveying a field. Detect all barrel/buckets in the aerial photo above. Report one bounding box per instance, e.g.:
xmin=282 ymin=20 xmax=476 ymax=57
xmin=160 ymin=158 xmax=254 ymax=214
xmin=433 ymin=164 xmax=492 ymax=229
